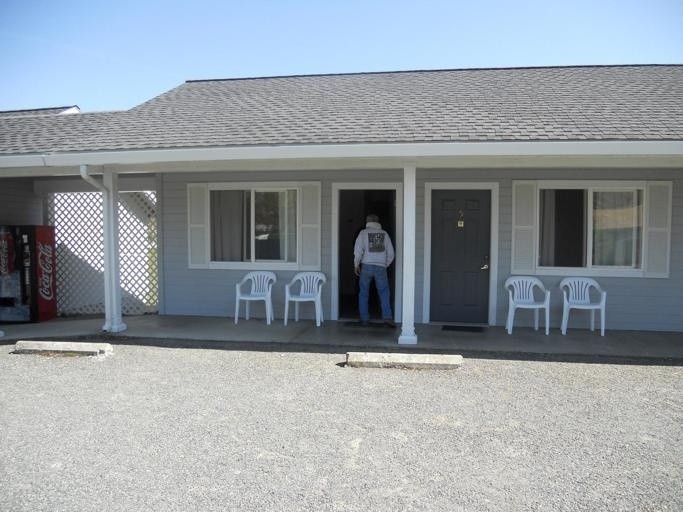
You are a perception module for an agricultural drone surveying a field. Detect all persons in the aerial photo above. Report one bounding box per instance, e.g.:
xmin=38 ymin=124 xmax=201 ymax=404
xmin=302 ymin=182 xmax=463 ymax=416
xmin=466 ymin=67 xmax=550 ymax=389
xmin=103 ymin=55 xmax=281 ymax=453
xmin=354 ymin=211 xmax=394 ymax=329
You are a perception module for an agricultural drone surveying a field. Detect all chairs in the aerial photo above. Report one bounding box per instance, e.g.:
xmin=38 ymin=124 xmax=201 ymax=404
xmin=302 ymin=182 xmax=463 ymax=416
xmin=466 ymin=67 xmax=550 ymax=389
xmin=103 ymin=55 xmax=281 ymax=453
xmin=557 ymin=277 xmax=605 ymax=337
xmin=502 ymin=276 xmax=549 ymax=335
xmin=232 ymin=270 xmax=278 ymax=326
xmin=283 ymin=271 xmax=326 ymax=328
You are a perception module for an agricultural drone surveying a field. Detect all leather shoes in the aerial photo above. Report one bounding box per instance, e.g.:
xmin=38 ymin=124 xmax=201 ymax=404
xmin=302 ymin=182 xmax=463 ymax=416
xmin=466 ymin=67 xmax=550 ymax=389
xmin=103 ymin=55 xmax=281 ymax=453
xmin=361 ymin=320 xmax=372 ymax=327
xmin=385 ymin=319 xmax=397 ymax=327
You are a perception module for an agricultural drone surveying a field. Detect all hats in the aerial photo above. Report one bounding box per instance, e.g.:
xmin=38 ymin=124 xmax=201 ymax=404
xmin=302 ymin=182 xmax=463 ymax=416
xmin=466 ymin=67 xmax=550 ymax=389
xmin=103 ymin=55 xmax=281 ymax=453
xmin=365 ymin=214 xmax=380 ymax=222
